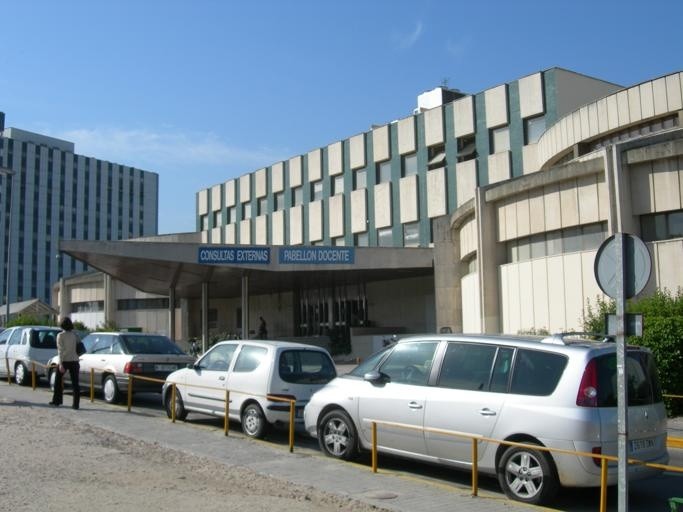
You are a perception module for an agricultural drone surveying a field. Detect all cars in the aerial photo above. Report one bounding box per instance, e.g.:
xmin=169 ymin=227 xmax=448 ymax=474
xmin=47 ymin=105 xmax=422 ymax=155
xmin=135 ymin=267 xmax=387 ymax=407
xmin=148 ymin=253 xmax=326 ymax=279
xmin=303 ymin=332 xmax=670 ymax=505
xmin=46 ymin=332 xmax=196 ymax=404
xmin=162 ymin=339 xmax=338 ymax=438
xmin=0 ymin=325 xmax=63 ymax=386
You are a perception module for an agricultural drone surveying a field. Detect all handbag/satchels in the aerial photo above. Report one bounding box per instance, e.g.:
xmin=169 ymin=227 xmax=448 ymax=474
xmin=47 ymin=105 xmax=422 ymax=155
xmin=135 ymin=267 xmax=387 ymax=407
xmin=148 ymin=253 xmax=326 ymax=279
xmin=76 ymin=342 xmax=87 ymax=356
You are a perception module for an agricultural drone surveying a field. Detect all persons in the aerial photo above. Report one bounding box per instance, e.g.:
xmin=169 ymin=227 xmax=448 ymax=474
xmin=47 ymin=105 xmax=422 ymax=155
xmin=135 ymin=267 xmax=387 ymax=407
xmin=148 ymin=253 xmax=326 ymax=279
xmin=260 ymin=316 xmax=267 ymax=339
xmin=190 ymin=340 xmax=200 ymax=357
xmin=49 ymin=316 xmax=81 ymax=409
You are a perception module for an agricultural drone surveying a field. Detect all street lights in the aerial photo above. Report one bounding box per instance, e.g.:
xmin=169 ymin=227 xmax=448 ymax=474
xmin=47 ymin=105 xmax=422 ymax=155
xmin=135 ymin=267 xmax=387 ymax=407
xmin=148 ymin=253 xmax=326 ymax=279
xmin=0 ymin=166 xmax=16 ymax=329
